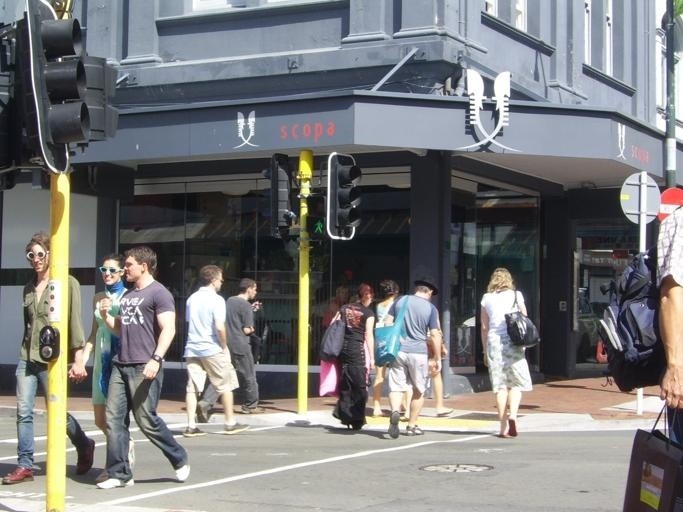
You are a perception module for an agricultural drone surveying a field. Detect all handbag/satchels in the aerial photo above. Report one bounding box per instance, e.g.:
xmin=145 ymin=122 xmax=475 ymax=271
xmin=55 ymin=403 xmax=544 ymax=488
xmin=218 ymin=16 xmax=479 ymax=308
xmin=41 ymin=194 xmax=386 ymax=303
xmin=251 ymin=333 xmax=264 ymax=363
xmin=319 ymin=321 xmax=346 ymax=362
xmin=506 ymin=311 xmax=540 ymax=346
xmin=319 ymin=360 xmax=342 ymax=398
xmin=598 ymin=246 xmax=666 ymax=391
xmin=373 ymin=326 xmax=408 ymax=367
xmin=623 ymin=400 xmax=682 ymax=512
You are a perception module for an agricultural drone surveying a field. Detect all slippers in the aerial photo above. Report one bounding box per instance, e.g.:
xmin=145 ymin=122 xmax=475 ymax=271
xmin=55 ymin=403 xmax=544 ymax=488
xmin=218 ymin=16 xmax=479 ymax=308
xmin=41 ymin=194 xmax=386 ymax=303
xmin=498 ymin=419 xmax=517 ymax=438
xmin=372 ymin=407 xmax=454 ymax=438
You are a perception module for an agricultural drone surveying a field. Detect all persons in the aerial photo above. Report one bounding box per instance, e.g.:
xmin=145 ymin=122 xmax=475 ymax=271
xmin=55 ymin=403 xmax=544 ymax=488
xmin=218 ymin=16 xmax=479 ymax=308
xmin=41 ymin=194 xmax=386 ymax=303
xmin=197 ymin=277 xmax=265 ymax=422
xmin=654 ymin=200 xmax=683 ymax=449
xmin=97 ymin=246 xmax=191 ymax=489
xmin=182 ymin=264 xmax=250 ymax=437
xmin=2 ymin=231 xmax=95 ymax=484
xmin=318 ymin=274 xmax=453 ymax=438
xmin=68 ymin=253 xmax=135 ymax=483
xmin=480 ymin=267 xmax=532 ymax=438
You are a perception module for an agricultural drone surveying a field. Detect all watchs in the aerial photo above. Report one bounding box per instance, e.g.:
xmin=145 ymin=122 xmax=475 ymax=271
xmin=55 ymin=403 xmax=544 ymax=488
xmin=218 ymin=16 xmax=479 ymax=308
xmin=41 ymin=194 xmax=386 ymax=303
xmin=150 ymin=354 xmax=162 ymax=362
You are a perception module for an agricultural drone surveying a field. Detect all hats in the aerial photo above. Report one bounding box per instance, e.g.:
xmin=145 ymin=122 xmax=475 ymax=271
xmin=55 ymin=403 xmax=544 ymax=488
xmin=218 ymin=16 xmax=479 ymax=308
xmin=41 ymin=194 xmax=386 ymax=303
xmin=414 ymin=276 xmax=439 ymax=296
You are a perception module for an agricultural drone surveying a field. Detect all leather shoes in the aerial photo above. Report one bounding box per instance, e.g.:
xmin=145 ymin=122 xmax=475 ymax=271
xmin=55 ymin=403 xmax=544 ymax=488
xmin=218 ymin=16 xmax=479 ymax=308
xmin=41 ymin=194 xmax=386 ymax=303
xmin=3 ymin=467 xmax=34 ymax=484
xmin=76 ymin=439 xmax=94 ymax=474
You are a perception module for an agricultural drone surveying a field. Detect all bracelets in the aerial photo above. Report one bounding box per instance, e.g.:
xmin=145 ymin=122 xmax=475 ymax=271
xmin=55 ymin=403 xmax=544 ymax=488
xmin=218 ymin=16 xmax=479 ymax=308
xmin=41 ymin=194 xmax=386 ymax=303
xmin=482 ymin=351 xmax=487 ymax=354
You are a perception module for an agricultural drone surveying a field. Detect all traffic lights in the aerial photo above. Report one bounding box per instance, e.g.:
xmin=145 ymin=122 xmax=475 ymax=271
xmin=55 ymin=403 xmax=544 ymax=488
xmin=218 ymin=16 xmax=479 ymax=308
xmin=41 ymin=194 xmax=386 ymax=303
xmin=14 ymin=0 xmax=90 ymax=176
xmin=305 ymin=194 xmax=327 ymax=242
xmin=261 ymin=151 xmax=291 ymax=243
xmin=76 ymin=51 xmax=121 ymax=144
xmin=0 ymin=21 xmax=23 ymax=190
xmin=326 ymin=152 xmax=362 ymax=241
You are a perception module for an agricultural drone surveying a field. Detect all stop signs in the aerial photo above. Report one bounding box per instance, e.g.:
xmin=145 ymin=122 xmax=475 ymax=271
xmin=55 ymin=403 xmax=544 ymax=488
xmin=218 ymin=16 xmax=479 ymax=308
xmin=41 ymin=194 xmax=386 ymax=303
xmin=657 ymin=187 xmax=682 ymax=222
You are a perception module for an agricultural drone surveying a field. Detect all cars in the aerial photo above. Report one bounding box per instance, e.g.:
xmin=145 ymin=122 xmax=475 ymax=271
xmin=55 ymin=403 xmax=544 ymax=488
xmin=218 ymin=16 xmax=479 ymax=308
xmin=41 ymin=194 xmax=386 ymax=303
xmin=458 ymin=283 xmax=603 ymax=365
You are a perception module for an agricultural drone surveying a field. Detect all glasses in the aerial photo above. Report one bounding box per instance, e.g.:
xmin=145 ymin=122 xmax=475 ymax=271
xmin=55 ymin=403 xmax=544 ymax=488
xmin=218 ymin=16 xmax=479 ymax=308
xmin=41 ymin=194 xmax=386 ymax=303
xmin=26 ymin=251 xmax=50 ymax=261
xmin=99 ymin=267 xmax=124 ymax=274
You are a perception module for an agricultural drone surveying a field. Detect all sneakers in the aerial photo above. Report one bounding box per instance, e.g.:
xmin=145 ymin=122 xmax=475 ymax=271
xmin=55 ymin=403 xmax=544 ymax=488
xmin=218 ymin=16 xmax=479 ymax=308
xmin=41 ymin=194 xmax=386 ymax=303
xmin=223 ymin=422 xmax=250 ymax=434
xmin=198 ymin=401 xmax=208 ymax=424
xmin=96 ymin=435 xmax=134 ymax=489
xmin=175 ymin=464 xmax=190 ymax=481
xmin=242 ymin=407 xmax=266 ymax=414
xmin=183 ymin=427 xmax=206 ymax=436
